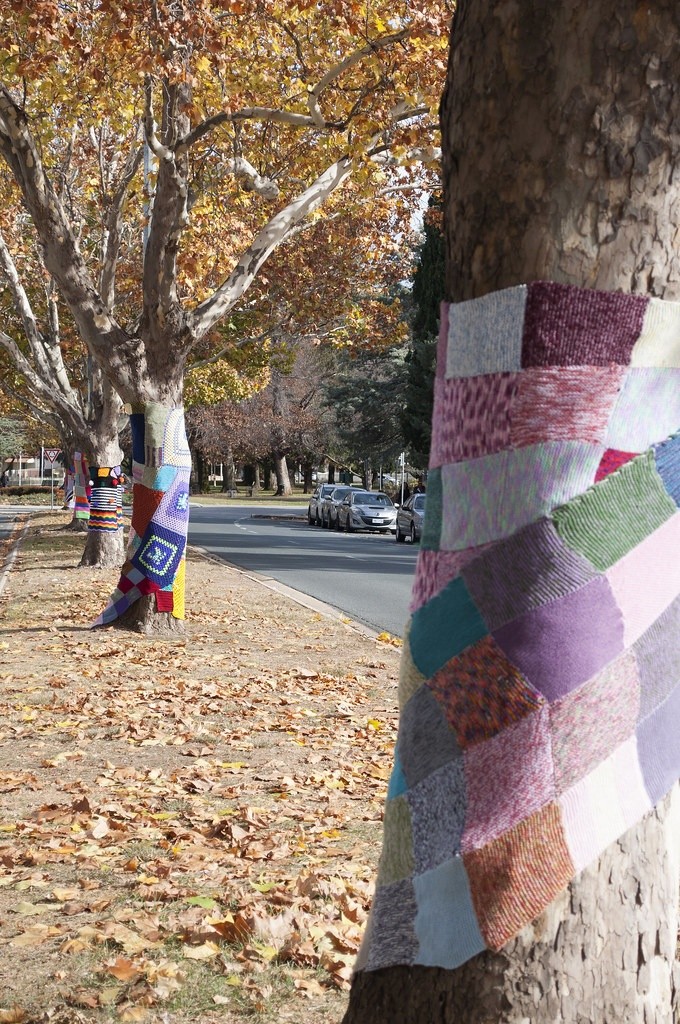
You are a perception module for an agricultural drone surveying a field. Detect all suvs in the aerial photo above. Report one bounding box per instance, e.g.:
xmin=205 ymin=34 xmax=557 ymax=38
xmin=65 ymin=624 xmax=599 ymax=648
xmin=307 ymin=482 xmax=350 ymax=527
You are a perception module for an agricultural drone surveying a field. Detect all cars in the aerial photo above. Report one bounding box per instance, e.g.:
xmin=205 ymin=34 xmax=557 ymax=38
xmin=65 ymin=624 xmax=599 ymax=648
xmin=335 ymin=490 xmax=399 ymax=536
xmin=321 ymin=487 xmax=377 ymax=530
xmin=376 ymin=475 xmax=401 ymax=487
xmin=394 ymin=492 xmax=425 ymax=545
